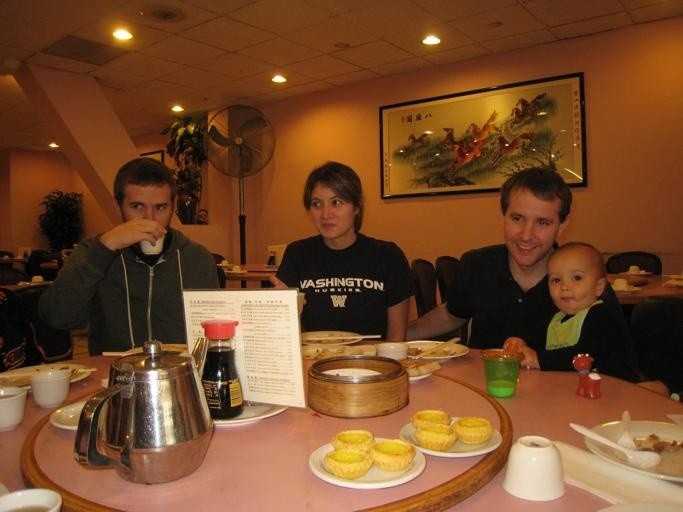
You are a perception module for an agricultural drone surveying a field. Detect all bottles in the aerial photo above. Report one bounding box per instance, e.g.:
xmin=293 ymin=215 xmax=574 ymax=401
xmin=201 ymin=320 xmax=245 ymax=419
xmin=267 ymin=251 xmax=276 ymax=267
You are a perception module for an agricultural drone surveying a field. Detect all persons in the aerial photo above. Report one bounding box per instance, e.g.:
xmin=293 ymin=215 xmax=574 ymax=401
xmin=405 ymin=167 xmax=669 ymax=396
xmin=274 ymin=161 xmax=415 ymax=342
xmin=498 ymin=242 xmax=618 ymax=371
xmin=37 ymin=157 xmax=220 ymax=354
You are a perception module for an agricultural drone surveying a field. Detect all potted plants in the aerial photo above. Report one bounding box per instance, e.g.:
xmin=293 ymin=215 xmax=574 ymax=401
xmin=160 ymin=110 xmax=212 ymax=224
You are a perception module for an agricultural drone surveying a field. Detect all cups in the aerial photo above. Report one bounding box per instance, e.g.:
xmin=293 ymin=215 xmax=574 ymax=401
xmin=139 ymin=232 xmax=166 ymax=255
xmin=31 ymin=276 xmax=45 ymax=285
xmin=216 ymin=259 xmax=248 ymax=274
xmin=505 ymin=436 xmax=566 ymax=502
xmin=480 ymin=353 xmax=524 ymax=398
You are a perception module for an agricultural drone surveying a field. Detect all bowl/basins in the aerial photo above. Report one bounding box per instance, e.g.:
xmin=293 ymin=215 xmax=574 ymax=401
xmin=2 ymin=486 xmax=63 ymax=512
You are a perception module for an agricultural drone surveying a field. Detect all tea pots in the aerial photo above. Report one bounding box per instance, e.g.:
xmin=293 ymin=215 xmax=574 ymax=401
xmin=74 ymin=337 xmax=215 ymax=486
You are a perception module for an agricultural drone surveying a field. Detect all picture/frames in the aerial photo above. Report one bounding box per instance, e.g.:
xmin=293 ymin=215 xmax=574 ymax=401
xmin=377 ymin=71 xmax=587 ymax=200
xmin=138 ymin=150 xmax=165 ymax=166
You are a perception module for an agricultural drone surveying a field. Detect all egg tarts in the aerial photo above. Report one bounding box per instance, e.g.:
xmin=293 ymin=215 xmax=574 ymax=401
xmin=321 ymin=409 xmax=493 ymax=480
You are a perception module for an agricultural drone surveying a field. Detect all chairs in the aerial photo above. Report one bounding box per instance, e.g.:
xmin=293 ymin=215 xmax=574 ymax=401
xmin=1 ymin=247 xmax=682 ymax=511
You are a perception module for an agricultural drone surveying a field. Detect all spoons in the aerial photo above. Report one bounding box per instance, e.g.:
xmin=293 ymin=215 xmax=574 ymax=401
xmin=568 ymin=408 xmax=662 ymax=468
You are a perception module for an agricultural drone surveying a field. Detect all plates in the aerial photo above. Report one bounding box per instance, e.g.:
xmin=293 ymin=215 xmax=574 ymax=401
xmin=0 ymin=363 xmax=98 ymax=433
xmin=306 ymin=415 xmax=504 ymax=492
xmin=211 ymin=403 xmax=290 ymax=427
xmin=301 ymin=329 xmax=470 ymax=381
xmin=587 ymin=418 xmax=683 ymax=483
xmin=98 ymin=342 xmax=189 ymax=356
xmin=612 ymin=264 xmax=683 ymax=293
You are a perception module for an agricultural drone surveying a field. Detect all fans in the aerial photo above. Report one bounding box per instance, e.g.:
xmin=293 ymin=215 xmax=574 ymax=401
xmin=199 ymin=103 xmax=276 ymax=287
